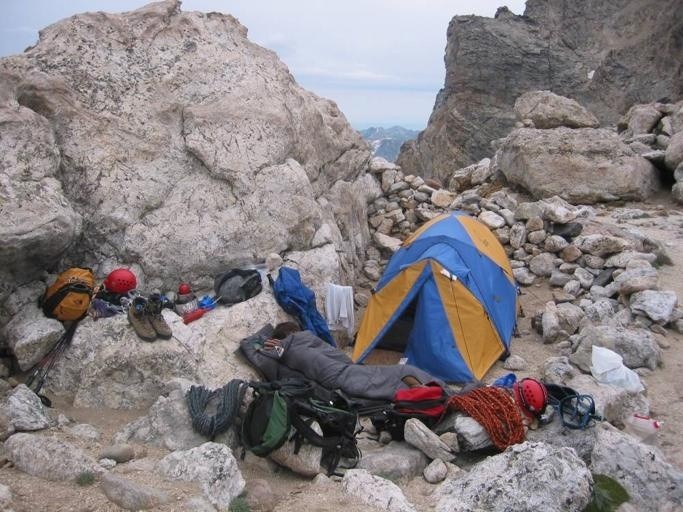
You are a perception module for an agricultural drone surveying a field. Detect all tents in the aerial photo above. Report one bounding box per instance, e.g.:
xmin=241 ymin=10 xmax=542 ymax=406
xmin=351 ymin=210 xmax=526 ymax=385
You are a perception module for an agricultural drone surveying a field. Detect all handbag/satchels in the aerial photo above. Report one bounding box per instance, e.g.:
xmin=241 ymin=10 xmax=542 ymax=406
xmin=215 ymin=267 xmax=263 ymax=304
xmin=393 ymin=386 xmax=446 ymax=422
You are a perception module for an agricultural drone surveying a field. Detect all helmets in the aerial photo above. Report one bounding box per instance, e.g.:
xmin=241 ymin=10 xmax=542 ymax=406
xmin=515 ymin=376 xmax=551 ymax=426
xmin=103 ymin=268 xmax=137 ymax=293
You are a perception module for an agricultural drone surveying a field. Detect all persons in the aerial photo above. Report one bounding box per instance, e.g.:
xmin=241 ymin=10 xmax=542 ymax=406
xmin=261 ymin=322 xmax=305 ymax=351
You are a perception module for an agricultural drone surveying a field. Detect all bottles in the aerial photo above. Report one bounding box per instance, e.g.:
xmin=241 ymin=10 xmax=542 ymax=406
xmin=628 ymin=414 xmax=660 ymax=432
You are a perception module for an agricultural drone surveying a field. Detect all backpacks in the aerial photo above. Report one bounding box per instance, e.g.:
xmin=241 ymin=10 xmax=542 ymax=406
xmin=38 ymin=265 xmax=95 ymax=322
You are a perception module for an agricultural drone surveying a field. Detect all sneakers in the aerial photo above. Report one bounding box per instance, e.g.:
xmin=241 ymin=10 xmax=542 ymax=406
xmin=145 ymin=294 xmax=173 ymax=340
xmin=126 ymin=296 xmax=157 ymax=343
